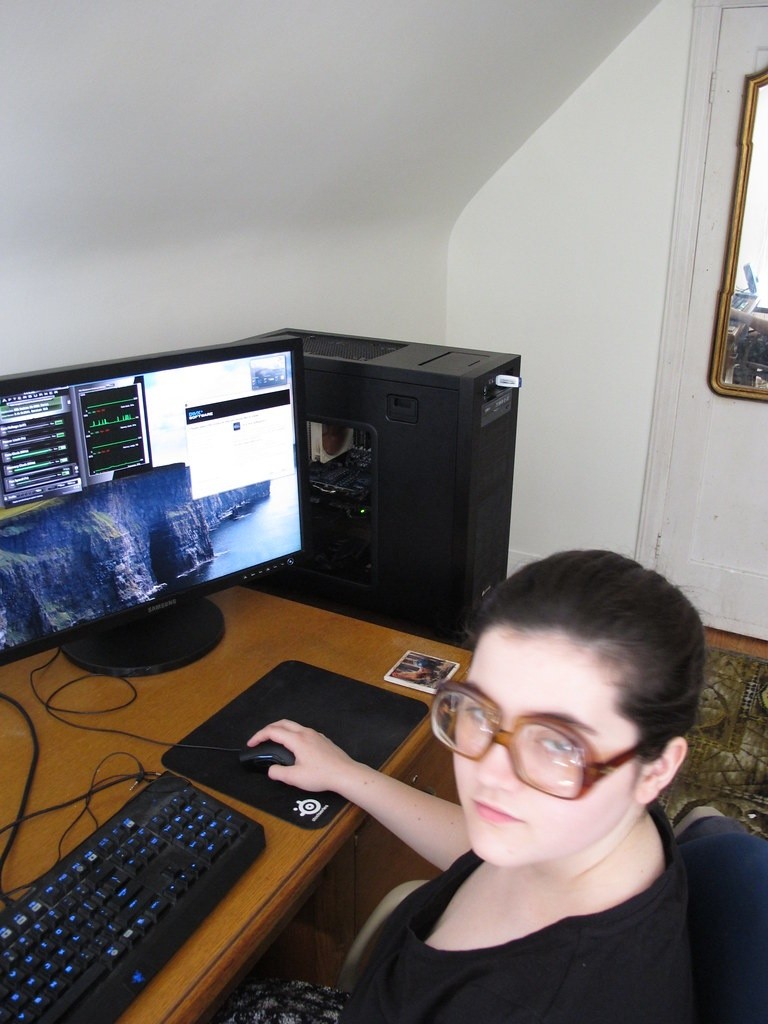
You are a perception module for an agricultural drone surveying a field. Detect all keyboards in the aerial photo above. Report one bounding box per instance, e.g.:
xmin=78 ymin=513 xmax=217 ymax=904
xmin=0 ymin=770 xmax=267 ymax=1024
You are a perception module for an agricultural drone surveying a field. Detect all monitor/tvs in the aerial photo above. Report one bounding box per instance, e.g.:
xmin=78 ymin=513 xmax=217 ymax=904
xmin=0 ymin=335 xmax=308 ymax=675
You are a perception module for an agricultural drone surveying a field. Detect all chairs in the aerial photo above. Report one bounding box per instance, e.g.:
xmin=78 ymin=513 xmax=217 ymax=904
xmin=339 ymin=806 xmax=768 ymax=1024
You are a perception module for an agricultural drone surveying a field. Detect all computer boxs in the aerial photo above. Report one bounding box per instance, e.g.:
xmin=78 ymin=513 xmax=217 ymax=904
xmin=242 ymin=327 xmax=522 ymax=648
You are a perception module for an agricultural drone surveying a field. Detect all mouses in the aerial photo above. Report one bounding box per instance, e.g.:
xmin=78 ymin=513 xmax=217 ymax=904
xmin=240 ymin=742 xmax=295 ymax=773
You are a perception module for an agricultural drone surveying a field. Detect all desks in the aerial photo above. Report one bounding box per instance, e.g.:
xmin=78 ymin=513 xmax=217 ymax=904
xmin=0 ymin=584 xmax=474 ymax=1024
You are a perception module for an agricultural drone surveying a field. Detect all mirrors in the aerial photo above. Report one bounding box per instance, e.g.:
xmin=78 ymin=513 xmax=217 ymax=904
xmin=706 ymin=66 xmax=768 ymax=404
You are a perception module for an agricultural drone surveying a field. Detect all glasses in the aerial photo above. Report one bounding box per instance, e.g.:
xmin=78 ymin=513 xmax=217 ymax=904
xmin=430 ymin=679 xmax=680 ymax=802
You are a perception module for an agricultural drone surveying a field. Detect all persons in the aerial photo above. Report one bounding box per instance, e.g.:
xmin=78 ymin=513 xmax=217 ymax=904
xmin=728 ymin=307 xmax=768 ymax=387
xmin=204 ymin=548 xmax=707 ymax=1024
